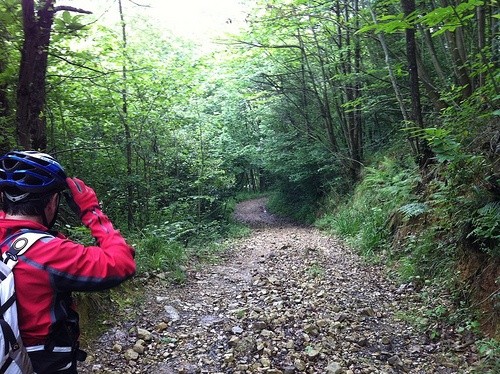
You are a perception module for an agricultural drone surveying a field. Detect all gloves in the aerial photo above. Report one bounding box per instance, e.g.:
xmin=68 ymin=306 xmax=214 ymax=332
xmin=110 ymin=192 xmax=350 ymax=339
xmin=64 ymin=176 xmax=101 ymax=219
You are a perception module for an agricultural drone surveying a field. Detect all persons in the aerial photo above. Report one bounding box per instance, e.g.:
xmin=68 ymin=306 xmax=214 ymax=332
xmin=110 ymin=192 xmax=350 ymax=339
xmin=0 ymin=150 xmax=137 ymax=374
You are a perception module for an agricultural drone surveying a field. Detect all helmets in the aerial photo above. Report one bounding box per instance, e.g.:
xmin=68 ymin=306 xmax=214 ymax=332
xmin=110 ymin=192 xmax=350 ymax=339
xmin=0 ymin=151 xmax=67 ymax=192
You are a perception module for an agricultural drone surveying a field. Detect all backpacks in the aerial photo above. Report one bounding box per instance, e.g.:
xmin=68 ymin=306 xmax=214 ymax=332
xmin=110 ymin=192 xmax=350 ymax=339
xmin=0 ymin=232 xmax=55 ymax=374
